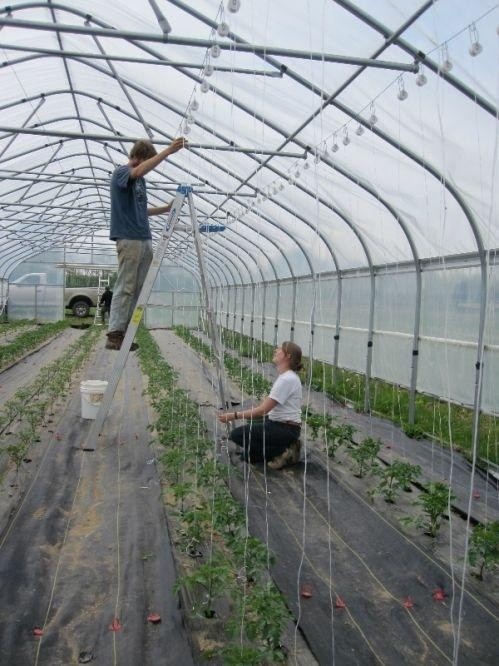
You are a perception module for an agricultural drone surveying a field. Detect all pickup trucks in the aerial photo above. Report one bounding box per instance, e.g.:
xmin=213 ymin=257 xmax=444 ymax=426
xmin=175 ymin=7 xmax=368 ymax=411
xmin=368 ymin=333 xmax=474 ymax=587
xmin=0 ymin=272 xmax=107 ymax=317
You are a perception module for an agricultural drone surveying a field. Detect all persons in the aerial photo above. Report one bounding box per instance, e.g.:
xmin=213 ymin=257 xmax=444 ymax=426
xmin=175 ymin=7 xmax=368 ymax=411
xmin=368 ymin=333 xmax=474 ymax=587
xmin=216 ymin=342 xmax=303 ymax=466
xmin=98 ymin=286 xmax=113 ymax=324
xmin=104 ymin=136 xmax=188 ymax=351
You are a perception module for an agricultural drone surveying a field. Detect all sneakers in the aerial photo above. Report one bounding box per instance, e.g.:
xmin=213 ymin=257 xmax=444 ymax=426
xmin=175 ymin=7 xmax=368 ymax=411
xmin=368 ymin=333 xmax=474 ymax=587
xmin=267 ymin=439 xmax=300 ymax=469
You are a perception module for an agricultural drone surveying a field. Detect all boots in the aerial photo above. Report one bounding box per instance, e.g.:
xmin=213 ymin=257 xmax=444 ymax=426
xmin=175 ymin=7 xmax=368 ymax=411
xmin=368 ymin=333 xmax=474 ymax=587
xmin=106 ymin=331 xmax=139 ymax=351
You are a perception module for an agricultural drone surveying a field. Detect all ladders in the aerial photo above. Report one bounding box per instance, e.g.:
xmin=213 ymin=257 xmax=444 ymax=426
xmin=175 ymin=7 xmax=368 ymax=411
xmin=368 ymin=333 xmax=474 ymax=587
xmin=81 ymin=183 xmax=236 ymax=452
xmin=92 ymin=275 xmax=111 ymax=327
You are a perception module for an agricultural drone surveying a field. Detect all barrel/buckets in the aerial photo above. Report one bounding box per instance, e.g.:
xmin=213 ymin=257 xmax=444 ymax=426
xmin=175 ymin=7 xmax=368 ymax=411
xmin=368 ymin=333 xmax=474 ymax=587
xmin=80 ymin=378 xmax=107 ymax=420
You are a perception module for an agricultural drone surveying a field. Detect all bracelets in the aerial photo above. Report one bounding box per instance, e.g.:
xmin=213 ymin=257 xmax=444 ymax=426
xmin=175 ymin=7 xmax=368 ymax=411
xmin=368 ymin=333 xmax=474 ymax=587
xmin=235 ymin=411 xmax=238 ymax=420
